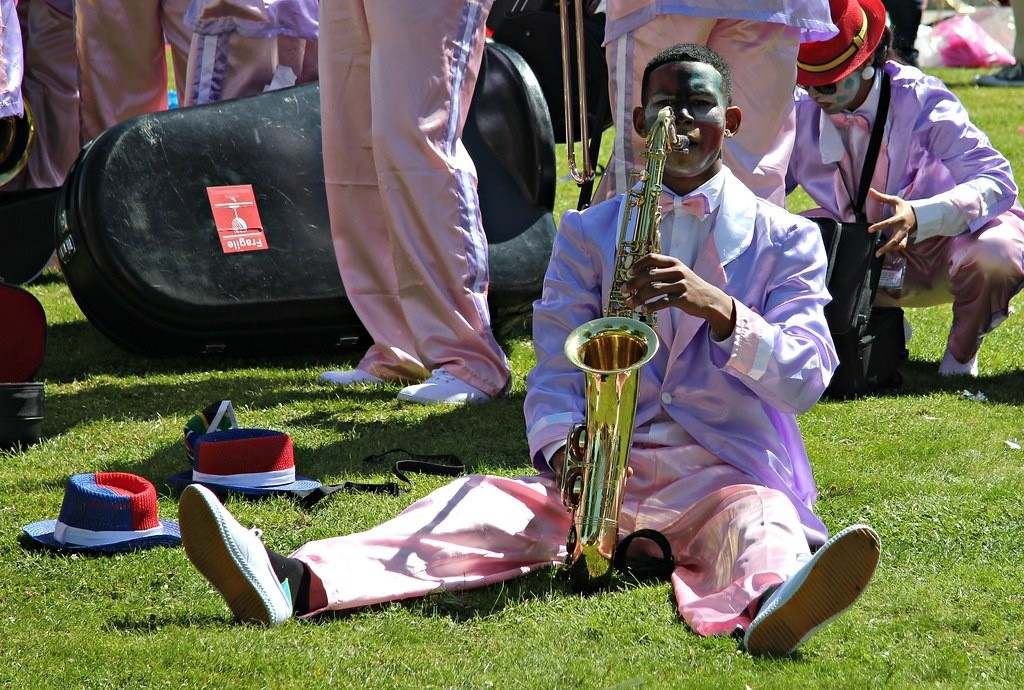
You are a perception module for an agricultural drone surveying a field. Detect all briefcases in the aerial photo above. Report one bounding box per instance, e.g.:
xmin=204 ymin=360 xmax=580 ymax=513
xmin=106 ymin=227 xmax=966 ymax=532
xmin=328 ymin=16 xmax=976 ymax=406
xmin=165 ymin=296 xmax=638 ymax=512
xmin=797 ymin=216 xmax=908 ymax=402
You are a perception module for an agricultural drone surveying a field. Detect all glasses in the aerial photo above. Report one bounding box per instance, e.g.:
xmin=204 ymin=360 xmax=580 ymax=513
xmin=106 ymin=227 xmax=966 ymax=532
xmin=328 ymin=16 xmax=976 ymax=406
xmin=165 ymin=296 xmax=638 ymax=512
xmin=796 ymin=83 xmax=838 ymax=95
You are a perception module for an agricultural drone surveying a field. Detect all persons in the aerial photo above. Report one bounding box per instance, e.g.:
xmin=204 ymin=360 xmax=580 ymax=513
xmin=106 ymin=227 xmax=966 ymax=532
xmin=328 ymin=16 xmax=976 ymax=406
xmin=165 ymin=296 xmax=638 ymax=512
xmin=316 ymin=1 xmax=515 ymax=407
xmin=603 ymin=0 xmax=840 ymax=209
xmin=16 ymin=1 xmax=320 ymax=190
xmin=179 ymin=45 xmax=880 ymax=662
xmin=789 ymin=0 xmax=1024 ymax=393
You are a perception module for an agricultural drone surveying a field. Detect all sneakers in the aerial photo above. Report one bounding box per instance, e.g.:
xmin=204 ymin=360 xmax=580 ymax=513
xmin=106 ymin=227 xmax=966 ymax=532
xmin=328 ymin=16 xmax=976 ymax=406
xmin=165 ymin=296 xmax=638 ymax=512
xmin=938 ymin=346 xmax=978 ymax=379
xmin=178 ymin=483 xmax=292 ymax=631
xmin=744 ymin=524 xmax=881 ymax=660
xmin=318 ymin=369 xmax=387 ymax=384
xmin=903 ymin=316 xmax=912 ymax=343
xmin=974 ymin=61 xmax=1024 ymax=86
xmin=396 ymin=364 xmax=510 ymax=404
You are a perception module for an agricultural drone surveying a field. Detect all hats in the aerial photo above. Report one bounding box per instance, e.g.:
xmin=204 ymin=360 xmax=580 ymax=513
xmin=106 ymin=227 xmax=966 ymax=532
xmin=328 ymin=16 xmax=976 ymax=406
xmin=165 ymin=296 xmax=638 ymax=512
xmin=23 ymin=473 xmax=183 ymax=550
xmin=796 ymin=0 xmax=887 ymax=86
xmin=166 ymin=428 xmax=322 ymax=493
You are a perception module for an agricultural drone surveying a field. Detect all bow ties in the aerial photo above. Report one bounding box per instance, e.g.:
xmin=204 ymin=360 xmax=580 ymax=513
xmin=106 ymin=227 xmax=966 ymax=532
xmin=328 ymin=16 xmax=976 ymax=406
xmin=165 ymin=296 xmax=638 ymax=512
xmin=829 ymin=109 xmax=874 ymax=133
xmin=658 ymin=190 xmax=711 ymax=222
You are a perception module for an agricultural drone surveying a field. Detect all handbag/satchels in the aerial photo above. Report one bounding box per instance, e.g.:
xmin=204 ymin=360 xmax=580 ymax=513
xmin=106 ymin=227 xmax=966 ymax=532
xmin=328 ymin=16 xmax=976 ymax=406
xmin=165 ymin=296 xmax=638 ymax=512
xmin=493 ymin=1 xmax=614 ymax=142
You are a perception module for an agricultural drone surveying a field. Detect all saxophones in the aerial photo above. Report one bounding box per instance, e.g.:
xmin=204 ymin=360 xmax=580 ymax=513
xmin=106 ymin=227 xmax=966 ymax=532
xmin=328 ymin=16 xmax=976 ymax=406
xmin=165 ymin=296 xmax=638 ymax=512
xmin=560 ymin=105 xmax=691 ymax=589
